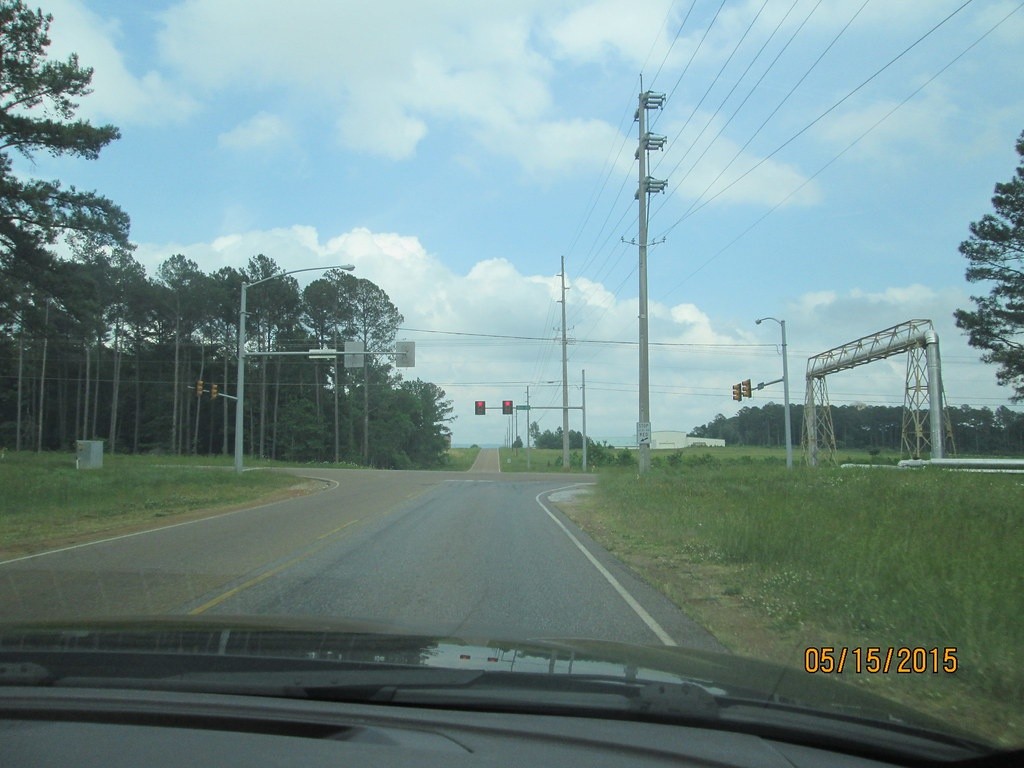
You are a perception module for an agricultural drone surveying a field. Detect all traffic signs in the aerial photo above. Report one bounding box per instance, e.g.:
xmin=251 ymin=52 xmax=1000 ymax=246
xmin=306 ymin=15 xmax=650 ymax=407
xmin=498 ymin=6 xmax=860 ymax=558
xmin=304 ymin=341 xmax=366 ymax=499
xmin=636 ymin=422 xmax=651 ymax=445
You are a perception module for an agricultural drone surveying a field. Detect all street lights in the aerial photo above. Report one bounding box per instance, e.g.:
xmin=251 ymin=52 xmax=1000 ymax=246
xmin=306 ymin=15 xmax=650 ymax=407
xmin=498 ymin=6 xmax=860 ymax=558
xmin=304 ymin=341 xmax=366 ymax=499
xmin=234 ymin=263 xmax=357 ymax=480
xmin=754 ymin=316 xmax=794 ymax=469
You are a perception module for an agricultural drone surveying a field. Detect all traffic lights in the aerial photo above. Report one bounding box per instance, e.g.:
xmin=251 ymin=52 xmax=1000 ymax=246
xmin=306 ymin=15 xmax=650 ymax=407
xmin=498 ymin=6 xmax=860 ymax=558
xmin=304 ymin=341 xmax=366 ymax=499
xmin=210 ymin=382 xmax=219 ymax=401
xmin=195 ymin=379 xmax=204 ymax=397
xmin=474 ymin=401 xmax=486 ymax=416
xmin=732 ymin=383 xmax=742 ymax=402
xmin=741 ymin=378 xmax=752 ymax=398
xmin=502 ymin=400 xmax=514 ymax=415
